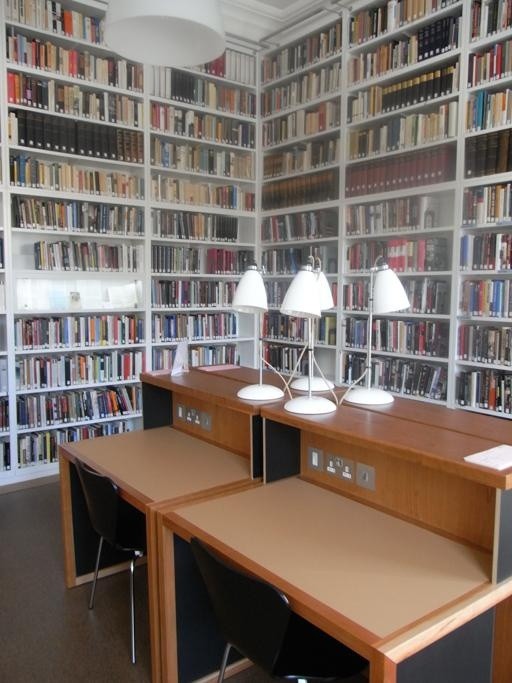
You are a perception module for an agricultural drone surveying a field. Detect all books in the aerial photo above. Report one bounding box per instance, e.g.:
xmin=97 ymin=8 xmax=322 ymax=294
xmin=3 ymin=1 xmax=512 ymax=471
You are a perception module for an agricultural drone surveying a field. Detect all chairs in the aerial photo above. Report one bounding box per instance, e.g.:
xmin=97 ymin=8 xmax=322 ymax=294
xmin=74 ymin=456 xmax=147 ymax=666
xmin=178 ymin=530 xmax=368 ymax=680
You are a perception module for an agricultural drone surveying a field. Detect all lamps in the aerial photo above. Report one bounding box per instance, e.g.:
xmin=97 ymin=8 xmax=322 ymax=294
xmin=231 ymin=264 xmax=284 ymax=401
xmin=99 ymin=0 xmax=226 ymax=70
xmin=280 ymin=265 xmax=338 ymax=417
xmin=341 ymin=254 xmax=412 ymax=406
xmin=287 ymin=262 xmax=336 ymax=393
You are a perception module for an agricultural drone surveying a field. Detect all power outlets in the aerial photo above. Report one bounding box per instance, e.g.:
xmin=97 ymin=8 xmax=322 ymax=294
xmin=185 ymin=408 xmax=200 ymax=424
xmin=325 ymin=454 xmax=355 ymax=481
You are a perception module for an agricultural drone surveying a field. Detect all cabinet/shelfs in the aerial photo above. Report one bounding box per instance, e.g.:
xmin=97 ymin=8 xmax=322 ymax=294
xmin=0 ymin=0 xmax=258 ymax=495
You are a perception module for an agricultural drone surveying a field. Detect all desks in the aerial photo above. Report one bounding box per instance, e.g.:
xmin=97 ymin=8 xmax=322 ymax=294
xmin=58 ymin=371 xmax=289 ymax=682
xmin=193 ymin=363 xmax=511 ymax=447
xmin=156 ymin=398 xmax=511 ymax=682
